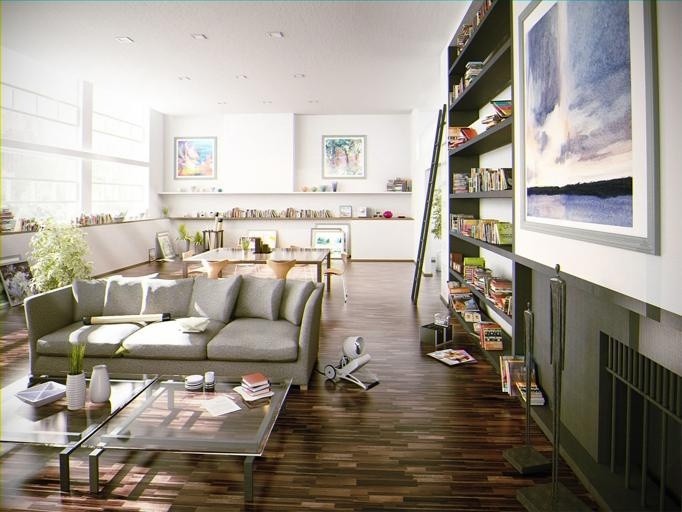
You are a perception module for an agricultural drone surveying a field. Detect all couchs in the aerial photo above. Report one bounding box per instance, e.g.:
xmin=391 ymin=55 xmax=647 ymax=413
xmin=23 ymin=280 xmax=325 ymax=391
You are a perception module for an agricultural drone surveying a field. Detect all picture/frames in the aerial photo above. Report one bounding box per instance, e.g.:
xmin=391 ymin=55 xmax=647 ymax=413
xmin=338 ymin=205 xmax=352 ymax=218
xmin=173 ymin=136 xmax=217 ymax=180
xmin=322 ymin=134 xmax=367 ymax=179
xmin=314 ymin=222 xmax=351 ymax=256
xmin=156 ymin=231 xmax=176 ymax=259
xmin=0 ymin=255 xmax=41 ymax=307
xmin=310 ymin=228 xmax=346 ymax=259
xmin=517 ymin=0 xmax=662 ymax=256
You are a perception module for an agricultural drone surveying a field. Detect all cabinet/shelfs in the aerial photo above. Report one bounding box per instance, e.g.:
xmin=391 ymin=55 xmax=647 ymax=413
xmin=157 ymin=192 xmax=416 ymax=222
xmin=447 ymin=0 xmax=532 ymax=374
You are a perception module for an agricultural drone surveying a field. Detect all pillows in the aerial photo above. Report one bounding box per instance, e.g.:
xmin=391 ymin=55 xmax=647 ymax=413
xmin=72 ymin=274 xmax=315 ymax=326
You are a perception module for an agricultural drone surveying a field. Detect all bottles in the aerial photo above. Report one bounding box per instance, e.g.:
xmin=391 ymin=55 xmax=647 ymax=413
xmin=184 ymin=374 xmax=204 ymax=392
xmin=90 ymin=364 xmax=111 ymax=403
xmin=205 ymin=370 xmax=215 ymax=392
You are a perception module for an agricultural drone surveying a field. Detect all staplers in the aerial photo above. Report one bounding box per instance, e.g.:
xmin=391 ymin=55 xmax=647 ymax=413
xmin=205 ymin=371 xmax=215 ymax=390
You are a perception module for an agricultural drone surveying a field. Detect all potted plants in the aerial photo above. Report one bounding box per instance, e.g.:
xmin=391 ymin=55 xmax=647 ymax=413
xmin=65 ymin=340 xmax=87 ymax=411
xmin=174 ymin=222 xmax=193 ymax=251
xmin=193 ymin=231 xmax=204 ymax=255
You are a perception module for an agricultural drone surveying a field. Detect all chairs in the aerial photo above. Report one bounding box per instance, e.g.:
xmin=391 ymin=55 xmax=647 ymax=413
xmin=323 ymin=252 xmax=349 ymax=304
xmin=200 ymin=258 xmax=229 ymax=280
xmin=265 ymin=258 xmax=297 ymax=281
xmin=182 ymin=249 xmax=203 ymax=278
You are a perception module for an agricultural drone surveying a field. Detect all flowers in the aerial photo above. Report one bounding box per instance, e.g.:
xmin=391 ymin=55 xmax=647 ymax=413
xmin=22 ymin=218 xmax=96 ymax=294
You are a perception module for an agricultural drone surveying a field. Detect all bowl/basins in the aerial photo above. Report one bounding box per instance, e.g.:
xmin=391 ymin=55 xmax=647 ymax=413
xmin=113 ymin=217 xmax=125 ymax=222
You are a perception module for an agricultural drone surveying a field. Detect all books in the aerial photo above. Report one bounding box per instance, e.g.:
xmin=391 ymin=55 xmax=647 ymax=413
xmin=423 ymin=0 xmax=548 ymax=409
xmin=1 ymin=208 xmax=40 ymax=232
xmin=225 ymin=207 xmax=333 ymax=219
xmin=386 ymin=176 xmax=412 ymax=191
xmin=74 ymin=213 xmax=112 ymax=227
xmin=241 ymin=373 xmax=272 ymax=397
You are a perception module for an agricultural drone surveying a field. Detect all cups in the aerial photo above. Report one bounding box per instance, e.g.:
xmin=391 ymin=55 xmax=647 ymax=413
xmin=252 ymin=238 xmax=270 ymax=253
xmin=302 ymin=181 xmax=338 ymax=192
